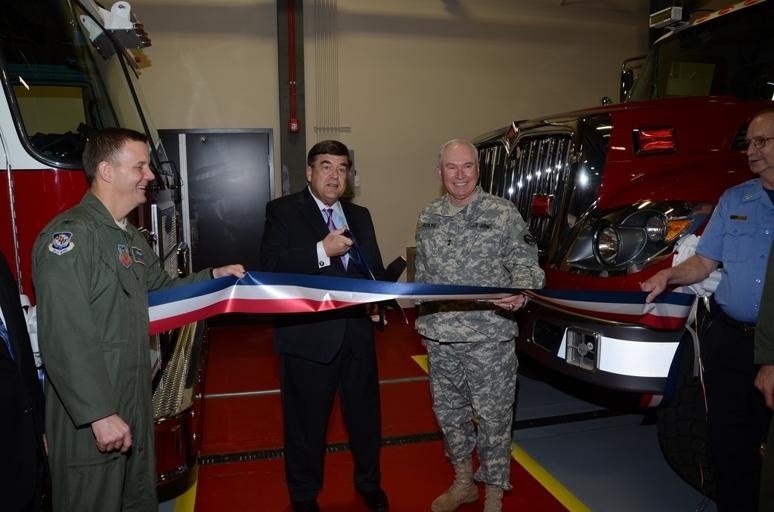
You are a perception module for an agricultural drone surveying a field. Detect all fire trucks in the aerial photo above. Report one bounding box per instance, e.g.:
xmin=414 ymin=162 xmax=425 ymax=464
xmin=469 ymin=1 xmax=773 ymax=426
xmin=0 ymin=0 xmax=213 ymax=512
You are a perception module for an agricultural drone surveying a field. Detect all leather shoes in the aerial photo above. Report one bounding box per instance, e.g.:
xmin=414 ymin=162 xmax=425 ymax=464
xmin=352 ymin=478 xmax=390 ymax=510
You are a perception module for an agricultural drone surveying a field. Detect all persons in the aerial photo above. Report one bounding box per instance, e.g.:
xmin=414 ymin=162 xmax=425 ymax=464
xmin=752 ymin=236 xmax=773 ymax=414
xmin=0 ymin=251 xmax=54 ymax=511
xmin=259 ymin=139 xmax=389 ymax=510
xmin=29 ymin=129 xmax=244 ymax=511
xmin=638 ymin=112 xmax=774 ymax=512
xmin=414 ymin=139 xmax=547 ymax=511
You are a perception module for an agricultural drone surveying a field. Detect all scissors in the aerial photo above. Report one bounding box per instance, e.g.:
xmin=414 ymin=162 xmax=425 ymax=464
xmin=344 ymin=229 xmax=408 ymax=331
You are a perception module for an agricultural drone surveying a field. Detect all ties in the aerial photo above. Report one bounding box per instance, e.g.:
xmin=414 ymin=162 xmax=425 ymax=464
xmin=324 ymin=209 xmax=338 ymax=238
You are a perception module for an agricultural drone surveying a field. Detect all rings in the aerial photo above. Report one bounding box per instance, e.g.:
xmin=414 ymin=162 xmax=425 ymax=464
xmin=509 ymin=302 xmax=515 ymax=310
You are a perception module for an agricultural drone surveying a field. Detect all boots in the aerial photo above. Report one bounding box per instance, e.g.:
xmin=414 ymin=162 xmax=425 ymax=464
xmin=431 ymin=456 xmax=506 ymax=511
